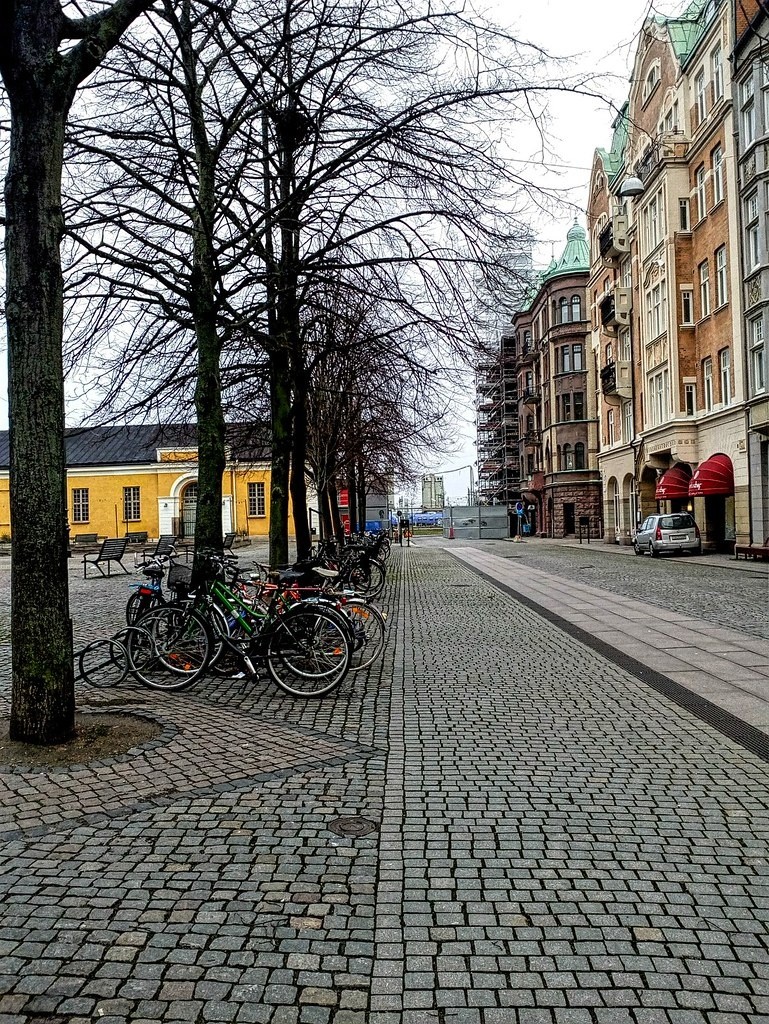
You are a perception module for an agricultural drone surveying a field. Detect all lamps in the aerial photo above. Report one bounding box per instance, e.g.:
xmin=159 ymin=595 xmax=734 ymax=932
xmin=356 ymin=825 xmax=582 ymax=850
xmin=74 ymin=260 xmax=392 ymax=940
xmin=687 ymin=500 xmax=694 ymax=514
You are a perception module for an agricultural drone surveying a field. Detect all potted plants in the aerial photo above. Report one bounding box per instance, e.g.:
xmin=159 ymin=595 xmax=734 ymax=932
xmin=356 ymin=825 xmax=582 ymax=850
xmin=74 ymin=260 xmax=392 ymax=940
xmin=0 ymin=534 xmax=11 ymax=554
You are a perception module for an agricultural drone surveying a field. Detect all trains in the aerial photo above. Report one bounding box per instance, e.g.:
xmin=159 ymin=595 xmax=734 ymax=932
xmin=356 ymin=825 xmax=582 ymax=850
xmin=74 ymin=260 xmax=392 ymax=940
xmin=392 ymin=512 xmax=443 ymax=527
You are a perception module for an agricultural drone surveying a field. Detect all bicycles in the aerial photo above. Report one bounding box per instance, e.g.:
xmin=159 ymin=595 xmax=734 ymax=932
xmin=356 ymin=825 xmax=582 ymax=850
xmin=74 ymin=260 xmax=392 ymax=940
xmin=127 ymin=529 xmax=392 ymax=701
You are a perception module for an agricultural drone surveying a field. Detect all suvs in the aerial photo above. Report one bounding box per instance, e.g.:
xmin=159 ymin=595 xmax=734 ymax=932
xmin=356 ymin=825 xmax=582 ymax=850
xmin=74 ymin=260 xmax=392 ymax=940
xmin=634 ymin=511 xmax=702 ymax=558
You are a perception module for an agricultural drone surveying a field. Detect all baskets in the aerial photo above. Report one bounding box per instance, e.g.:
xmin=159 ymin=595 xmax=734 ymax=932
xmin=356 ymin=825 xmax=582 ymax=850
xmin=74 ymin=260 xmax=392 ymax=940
xmin=167 ymin=553 xmax=195 ymax=590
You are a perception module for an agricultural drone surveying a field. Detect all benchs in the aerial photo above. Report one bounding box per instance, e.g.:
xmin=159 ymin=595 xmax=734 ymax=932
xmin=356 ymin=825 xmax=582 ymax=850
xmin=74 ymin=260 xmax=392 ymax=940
xmin=140 ymin=536 xmax=178 ymax=568
xmin=222 ymin=531 xmax=238 ymax=556
xmin=124 ymin=531 xmax=149 ymax=552
xmin=72 ymin=533 xmax=99 ymax=554
xmin=82 ymin=537 xmax=133 ymax=579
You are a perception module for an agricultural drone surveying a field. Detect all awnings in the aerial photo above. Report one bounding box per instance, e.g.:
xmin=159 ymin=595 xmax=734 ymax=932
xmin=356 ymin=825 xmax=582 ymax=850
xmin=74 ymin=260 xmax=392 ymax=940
xmin=688 ymin=455 xmax=734 ymax=497
xmin=654 ymin=463 xmax=693 ymax=500
